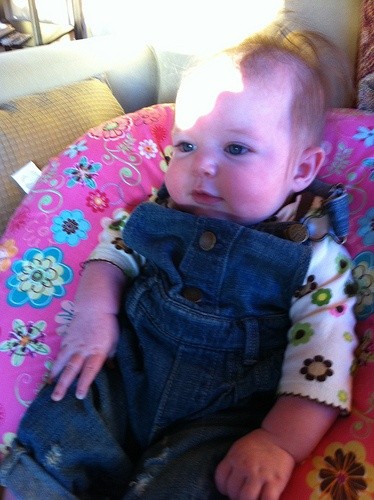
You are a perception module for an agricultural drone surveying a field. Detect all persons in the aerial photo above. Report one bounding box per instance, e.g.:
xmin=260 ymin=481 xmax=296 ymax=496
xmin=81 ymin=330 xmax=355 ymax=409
xmin=0 ymin=35 xmax=357 ymax=500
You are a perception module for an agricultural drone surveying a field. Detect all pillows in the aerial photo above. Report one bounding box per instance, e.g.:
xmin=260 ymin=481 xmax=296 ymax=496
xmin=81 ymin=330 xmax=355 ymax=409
xmin=0 ymin=72 xmax=126 ymax=238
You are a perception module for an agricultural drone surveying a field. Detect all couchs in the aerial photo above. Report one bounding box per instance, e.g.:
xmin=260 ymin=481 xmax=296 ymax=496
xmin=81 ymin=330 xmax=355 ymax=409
xmin=0 ymin=103 xmax=373 ymax=500
